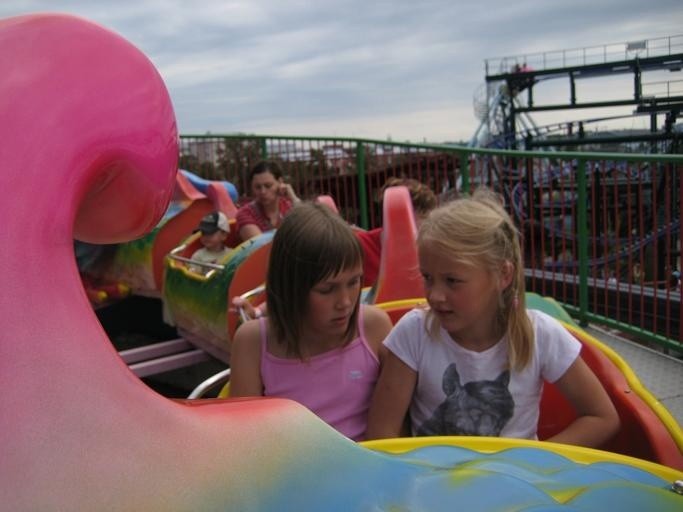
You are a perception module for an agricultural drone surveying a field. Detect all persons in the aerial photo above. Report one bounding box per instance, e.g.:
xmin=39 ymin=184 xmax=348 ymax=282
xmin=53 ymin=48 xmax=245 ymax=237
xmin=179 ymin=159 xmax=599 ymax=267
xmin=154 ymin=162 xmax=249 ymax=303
xmin=191 ymin=210 xmax=234 ymax=275
xmin=235 ymin=161 xmax=301 ymax=241
xmin=229 ymin=201 xmax=394 ymax=441
xmin=354 ymin=177 xmax=437 ymax=288
xmin=366 ymin=195 xmax=620 ymax=448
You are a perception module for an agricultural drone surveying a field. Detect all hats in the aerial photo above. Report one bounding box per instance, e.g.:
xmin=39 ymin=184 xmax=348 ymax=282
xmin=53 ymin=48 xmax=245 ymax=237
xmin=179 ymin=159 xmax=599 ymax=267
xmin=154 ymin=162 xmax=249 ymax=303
xmin=191 ymin=211 xmax=230 ymax=234
